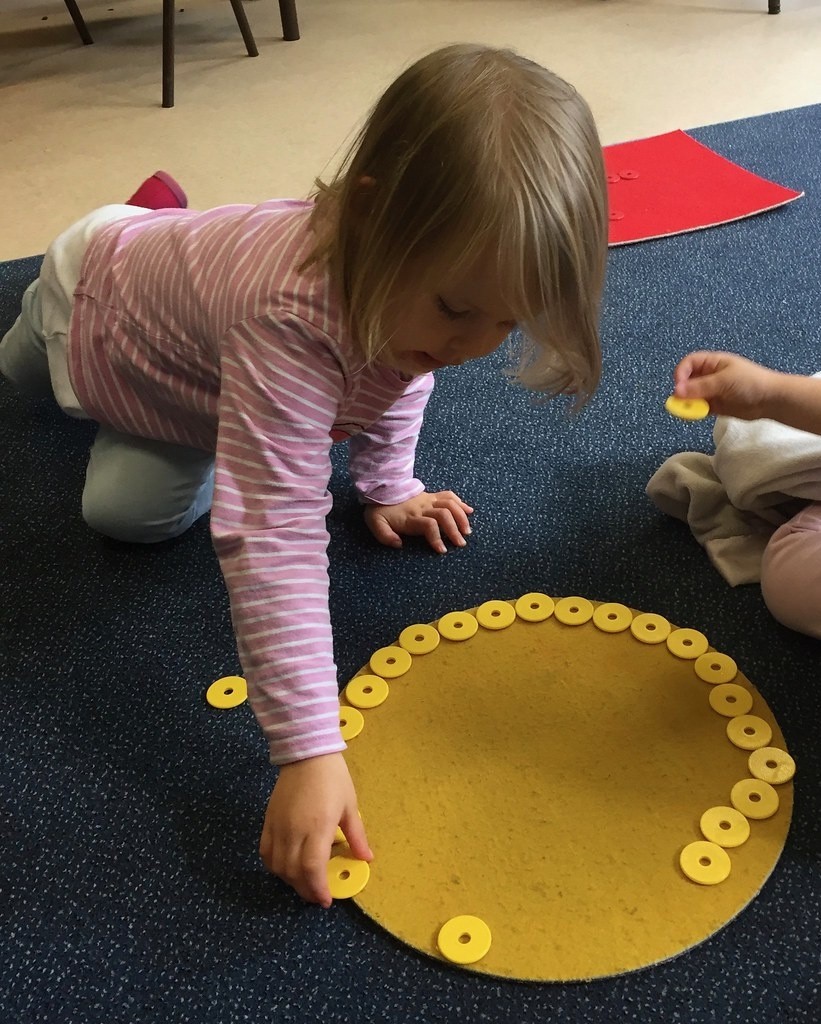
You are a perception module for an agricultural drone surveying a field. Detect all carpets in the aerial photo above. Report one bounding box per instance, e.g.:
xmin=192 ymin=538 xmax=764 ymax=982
xmin=0 ymin=101 xmax=821 ymax=1024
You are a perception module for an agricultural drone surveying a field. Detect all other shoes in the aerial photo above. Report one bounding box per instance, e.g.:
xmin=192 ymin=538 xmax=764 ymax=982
xmin=125 ymin=170 xmax=187 ymax=211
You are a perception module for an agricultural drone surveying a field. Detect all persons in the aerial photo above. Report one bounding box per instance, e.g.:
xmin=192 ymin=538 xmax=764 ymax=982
xmin=2 ymin=42 xmax=611 ymax=909
xmin=676 ymin=349 xmax=821 ymax=632
